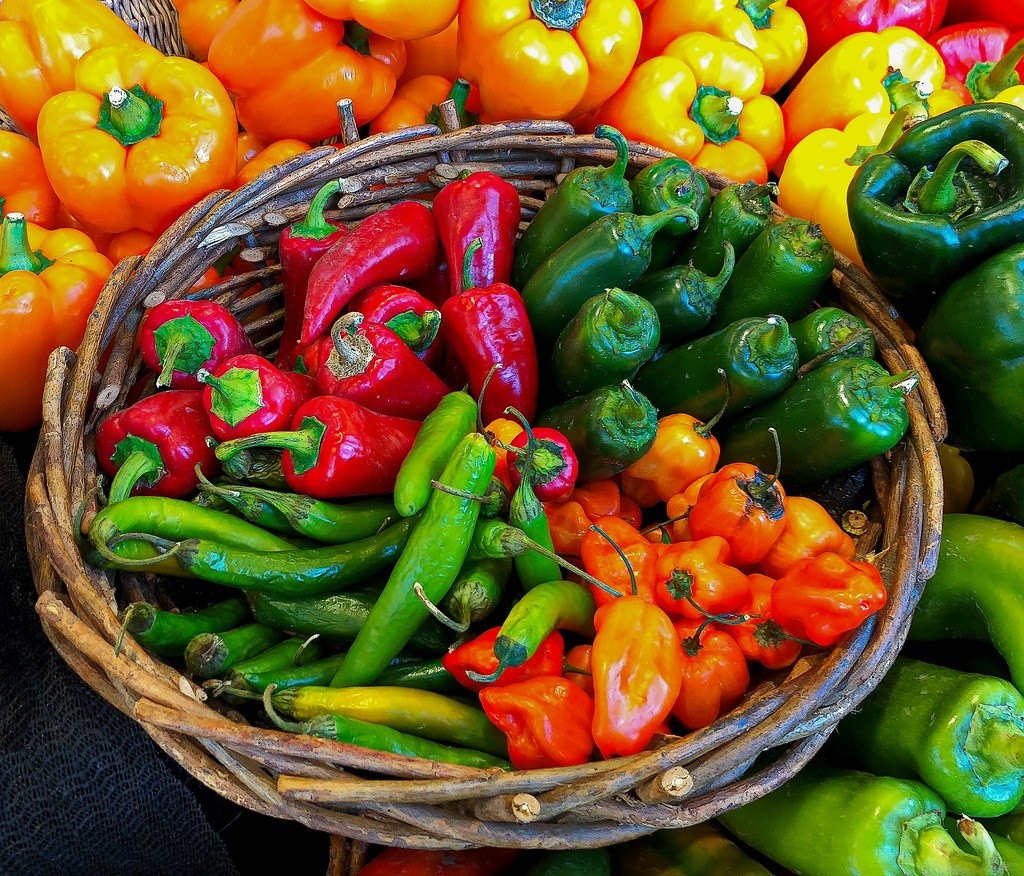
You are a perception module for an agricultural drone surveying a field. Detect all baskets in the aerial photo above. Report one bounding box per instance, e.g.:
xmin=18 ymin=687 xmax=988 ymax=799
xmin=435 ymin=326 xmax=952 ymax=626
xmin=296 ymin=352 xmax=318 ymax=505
xmin=23 ymin=100 xmax=947 ymax=876
xmin=99 ymin=0 xmax=194 ymax=60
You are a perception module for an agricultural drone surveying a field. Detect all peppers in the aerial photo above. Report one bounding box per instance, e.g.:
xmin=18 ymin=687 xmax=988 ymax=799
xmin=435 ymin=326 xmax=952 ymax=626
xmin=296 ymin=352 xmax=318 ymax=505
xmin=0 ymin=0 xmax=1024 ymax=876
